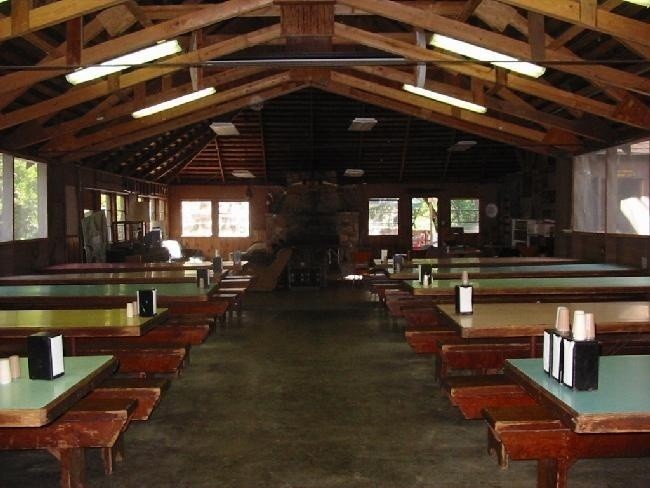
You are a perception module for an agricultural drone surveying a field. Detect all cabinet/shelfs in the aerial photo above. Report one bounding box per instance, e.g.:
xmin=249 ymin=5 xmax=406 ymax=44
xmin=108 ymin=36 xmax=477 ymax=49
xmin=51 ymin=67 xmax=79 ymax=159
xmin=512 ymin=218 xmax=537 ymax=247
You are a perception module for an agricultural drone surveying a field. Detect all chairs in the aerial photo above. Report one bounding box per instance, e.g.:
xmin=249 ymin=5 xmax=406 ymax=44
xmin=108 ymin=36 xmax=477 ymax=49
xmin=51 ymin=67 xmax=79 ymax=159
xmin=244 ymin=247 xmax=293 ymax=292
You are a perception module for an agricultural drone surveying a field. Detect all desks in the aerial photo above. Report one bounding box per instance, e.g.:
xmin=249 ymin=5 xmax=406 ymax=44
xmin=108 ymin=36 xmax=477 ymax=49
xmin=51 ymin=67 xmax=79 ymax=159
xmin=446 ymin=249 xmax=481 ymax=257
xmin=373 ymin=257 xmax=586 ymax=270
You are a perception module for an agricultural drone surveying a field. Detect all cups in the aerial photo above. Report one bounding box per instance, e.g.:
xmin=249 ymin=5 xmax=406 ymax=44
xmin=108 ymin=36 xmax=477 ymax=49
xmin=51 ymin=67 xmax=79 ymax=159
xmin=573 ymin=310 xmax=596 ymax=342
xmin=126 ymin=301 xmax=138 ymax=317
xmin=556 ymin=306 xmax=570 ymax=333
xmin=1 ymin=355 xmax=21 ymax=384
xmin=423 ymin=274 xmax=429 ymax=287
xmin=462 ymin=271 xmax=468 ymax=285
xmin=381 ymin=250 xmax=387 ymax=262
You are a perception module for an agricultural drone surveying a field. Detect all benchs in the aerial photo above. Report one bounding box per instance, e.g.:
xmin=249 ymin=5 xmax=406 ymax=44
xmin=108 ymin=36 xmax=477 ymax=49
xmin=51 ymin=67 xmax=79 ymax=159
xmin=355 ymin=264 xmax=650 ymax=488
xmin=0 ymin=261 xmax=254 ymax=488
